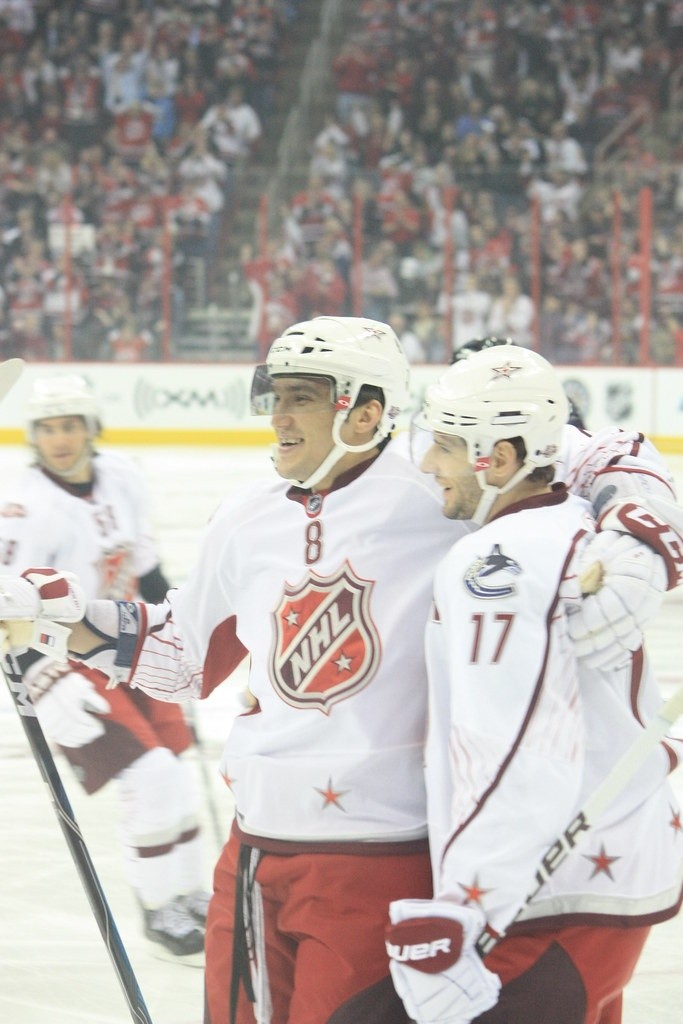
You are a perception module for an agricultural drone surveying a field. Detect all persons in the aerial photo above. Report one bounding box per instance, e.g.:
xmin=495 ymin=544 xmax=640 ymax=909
xmin=387 ymin=335 xmax=682 ymax=1024
xmin=2 ymin=381 xmax=216 ymax=967
xmin=0 ymin=0 xmax=683 ymax=364
xmin=0 ymin=317 xmax=681 ymax=1023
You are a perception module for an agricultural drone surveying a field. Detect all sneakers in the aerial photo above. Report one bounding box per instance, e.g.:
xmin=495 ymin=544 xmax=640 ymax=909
xmin=180 ymin=880 xmax=214 ymax=919
xmin=143 ymin=907 xmax=208 ymax=968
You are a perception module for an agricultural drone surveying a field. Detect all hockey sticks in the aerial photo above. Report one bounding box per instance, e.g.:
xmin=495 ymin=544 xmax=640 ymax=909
xmin=186 ymin=702 xmax=225 ymax=852
xmin=2 ymin=645 xmax=154 ymax=1024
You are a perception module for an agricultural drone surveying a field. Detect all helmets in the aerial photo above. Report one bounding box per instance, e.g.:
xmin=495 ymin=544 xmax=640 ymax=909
xmin=451 ymin=338 xmax=515 ymax=362
xmin=24 ymin=378 xmax=97 ymax=446
xmin=265 ymin=317 xmax=408 ymax=435
xmin=414 ymin=345 xmax=572 ymax=469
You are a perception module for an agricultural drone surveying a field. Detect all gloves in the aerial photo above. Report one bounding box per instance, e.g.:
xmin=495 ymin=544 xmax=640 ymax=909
xmin=566 ymin=506 xmax=683 ymax=672
xmin=0 ymin=569 xmax=85 ymax=664
xmin=383 ymin=899 xmax=501 ymax=1023
xmin=14 ymin=647 xmax=110 ymax=749
